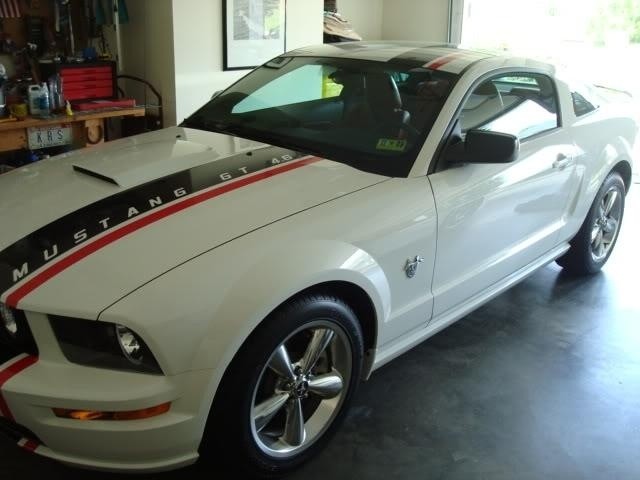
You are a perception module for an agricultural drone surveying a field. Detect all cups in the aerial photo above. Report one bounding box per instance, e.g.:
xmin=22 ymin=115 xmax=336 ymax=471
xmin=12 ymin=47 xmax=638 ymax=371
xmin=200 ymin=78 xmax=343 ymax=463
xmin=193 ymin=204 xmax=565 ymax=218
xmin=9 ymin=103 xmax=27 ymax=122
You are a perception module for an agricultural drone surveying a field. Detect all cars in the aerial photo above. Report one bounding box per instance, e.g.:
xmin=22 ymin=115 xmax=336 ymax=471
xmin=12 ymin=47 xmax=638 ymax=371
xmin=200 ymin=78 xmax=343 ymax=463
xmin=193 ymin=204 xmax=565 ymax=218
xmin=0 ymin=41 xmax=640 ymax=480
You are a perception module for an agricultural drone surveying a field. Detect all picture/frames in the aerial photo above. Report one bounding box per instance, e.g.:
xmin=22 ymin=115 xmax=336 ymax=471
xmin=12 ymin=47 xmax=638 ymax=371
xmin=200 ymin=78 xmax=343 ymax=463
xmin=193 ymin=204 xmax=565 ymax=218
xmin=222 ymin=0 xmax=288 ymax=72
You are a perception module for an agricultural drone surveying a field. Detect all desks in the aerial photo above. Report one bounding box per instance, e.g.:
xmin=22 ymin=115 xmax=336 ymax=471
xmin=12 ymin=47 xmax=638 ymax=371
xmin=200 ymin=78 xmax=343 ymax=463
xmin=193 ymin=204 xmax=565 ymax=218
xmin=0 ymin=107 xmax=145 ymax=153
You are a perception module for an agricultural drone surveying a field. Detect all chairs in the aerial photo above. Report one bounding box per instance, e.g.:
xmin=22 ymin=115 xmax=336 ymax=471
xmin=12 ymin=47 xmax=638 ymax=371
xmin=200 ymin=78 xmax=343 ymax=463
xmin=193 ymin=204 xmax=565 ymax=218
xmin=346 ymin=67 xmax=540 ymax=157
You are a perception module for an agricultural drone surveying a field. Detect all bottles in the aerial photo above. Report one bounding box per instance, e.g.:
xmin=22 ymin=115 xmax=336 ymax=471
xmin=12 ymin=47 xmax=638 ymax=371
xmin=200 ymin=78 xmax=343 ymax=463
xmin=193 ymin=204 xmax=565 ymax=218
xmin=40 ymin=82 xmax=50 ymax=117
